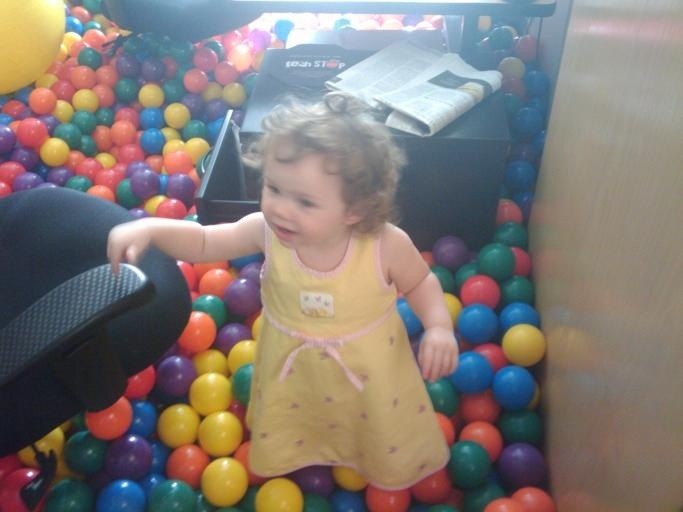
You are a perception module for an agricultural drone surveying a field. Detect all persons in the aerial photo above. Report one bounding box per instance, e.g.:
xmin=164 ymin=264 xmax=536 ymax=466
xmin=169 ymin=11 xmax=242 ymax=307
xmin=110 ymin=89 xmax=459 ymax=488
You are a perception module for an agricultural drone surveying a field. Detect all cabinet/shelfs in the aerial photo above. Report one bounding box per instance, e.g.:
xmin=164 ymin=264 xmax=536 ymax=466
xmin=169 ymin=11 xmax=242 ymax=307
xmin=187 ymin=45 xmax=514 ymax=258
